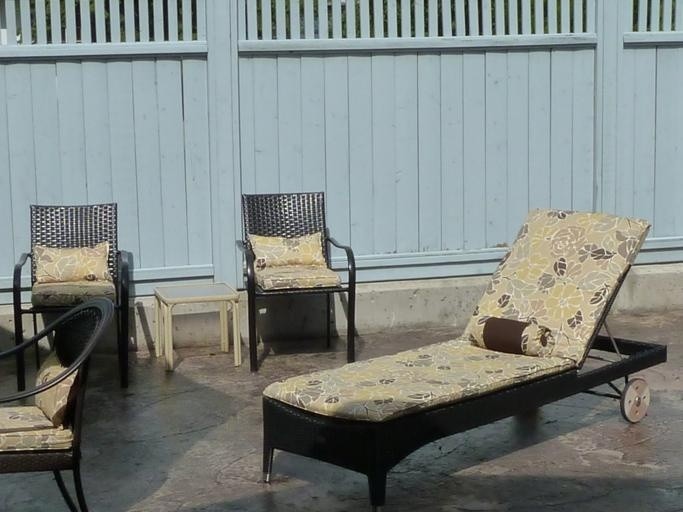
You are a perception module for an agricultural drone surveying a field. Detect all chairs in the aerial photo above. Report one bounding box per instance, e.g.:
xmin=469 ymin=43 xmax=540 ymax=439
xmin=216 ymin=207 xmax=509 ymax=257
xmin=259 ymin=205 xmax=670 ymax=508
xmin=13 ymin=203 xmax=128 ymax=400
xmin=234 ymin=193 xmax=358 ymax=372
xmin=0 ymin=294 xmax=117 ymax=511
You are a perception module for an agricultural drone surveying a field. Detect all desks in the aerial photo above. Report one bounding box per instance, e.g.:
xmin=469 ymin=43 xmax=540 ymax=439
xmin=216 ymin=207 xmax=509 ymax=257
xmin=153 ymin=280 xmax=241 ymax=371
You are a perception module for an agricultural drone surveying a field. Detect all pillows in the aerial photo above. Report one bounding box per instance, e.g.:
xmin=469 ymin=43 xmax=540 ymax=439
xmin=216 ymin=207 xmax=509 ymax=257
xmin=32 ymin=363 xmax=78 ymax=427
xmin=247 ymin=230 xmax=325 ymax=270
xmin=31 ymin=237 xmax=114 ymax=285
xmin=467 ymin=314 xmax=556 ymax=360
